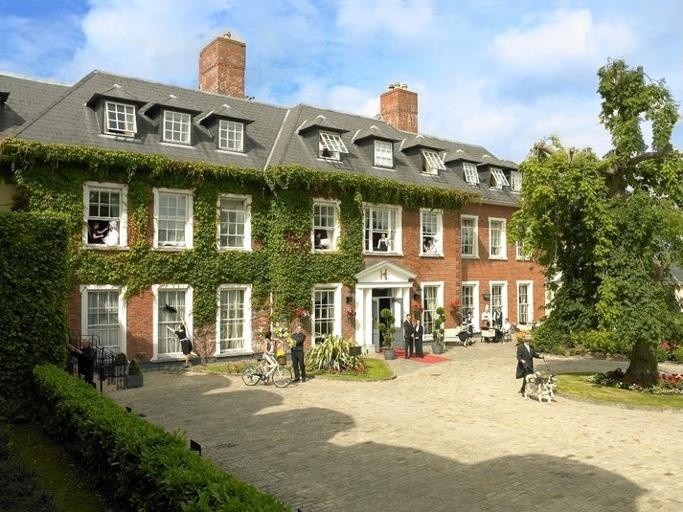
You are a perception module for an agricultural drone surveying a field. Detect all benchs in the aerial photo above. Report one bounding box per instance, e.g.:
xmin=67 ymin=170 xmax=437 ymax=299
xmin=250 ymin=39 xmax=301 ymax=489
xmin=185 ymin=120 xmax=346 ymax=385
xmin=444 ymin=328 xmax=470 ymax=346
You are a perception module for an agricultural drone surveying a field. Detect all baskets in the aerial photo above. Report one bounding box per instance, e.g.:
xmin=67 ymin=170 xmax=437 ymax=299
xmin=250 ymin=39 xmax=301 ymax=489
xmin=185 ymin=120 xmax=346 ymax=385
xmin=278 ymin=356 xmax=287 ymax=365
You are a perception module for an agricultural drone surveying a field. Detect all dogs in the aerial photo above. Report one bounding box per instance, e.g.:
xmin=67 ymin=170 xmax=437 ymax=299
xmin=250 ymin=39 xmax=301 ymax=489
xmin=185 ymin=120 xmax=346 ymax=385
xmin=523 ymin=372 xmax=558 ymax=404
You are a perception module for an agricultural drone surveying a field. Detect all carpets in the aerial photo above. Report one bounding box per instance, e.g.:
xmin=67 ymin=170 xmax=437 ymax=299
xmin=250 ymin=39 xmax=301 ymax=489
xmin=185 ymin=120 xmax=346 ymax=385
xmin=379 ymin=345 xmax=449 ymax=364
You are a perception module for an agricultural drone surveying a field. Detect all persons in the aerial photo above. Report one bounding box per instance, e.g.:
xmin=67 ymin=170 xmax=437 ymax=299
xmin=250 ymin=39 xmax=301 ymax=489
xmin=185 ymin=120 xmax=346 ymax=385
xmin=166 ymin=315 xmax=200 ymax=370
xmin=455 ymin=304 xmax=513 ymax=347
xmin=422 ymin=236 xmax=439 ymax=255
xmin=69 ymin=337 xmax=96 ymax=391
xmin=260 ymin=330 xmax=283 ymax=386
xmin=402 ymin=314 xmax=415 ymax=359
xmin=101 ymin=221 xmax=118 ymax=246
xmin=376 ymin=232 xmax=391 ymax=252
xmin=314 ymin=230 xmax=325 ymax=250
xmin=413 ymin=318 xmax=425 ymax=358
xmin=287 ymin=324 xmax=306 ymax=382
xmin=514 ymin=333 xmax=543 ymax=398
xmin=88 ymin=221 xmax=108 ymax=243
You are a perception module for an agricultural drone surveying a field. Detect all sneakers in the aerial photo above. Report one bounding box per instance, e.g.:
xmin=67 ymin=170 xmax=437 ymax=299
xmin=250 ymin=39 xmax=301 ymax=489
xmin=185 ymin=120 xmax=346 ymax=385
xmin=293 ymin=379 xmax=305 ymax=382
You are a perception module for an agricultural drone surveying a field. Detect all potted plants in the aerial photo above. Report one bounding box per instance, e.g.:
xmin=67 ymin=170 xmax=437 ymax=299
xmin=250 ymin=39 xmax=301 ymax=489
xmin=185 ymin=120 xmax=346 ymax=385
xmin=126 ymin=360 xmax=143 ymax=388
xmin=432 ymin=307 xmax=444 ymax=354
xmin=343 ymin=306 xmax=355 ymax=324
xmin=112 ymin=353 xmax=128 ymax=377
xmin=375 ymin=308 xmax=395 ymax=360
xmin=412 ymin=302 xmax=423 ymax=320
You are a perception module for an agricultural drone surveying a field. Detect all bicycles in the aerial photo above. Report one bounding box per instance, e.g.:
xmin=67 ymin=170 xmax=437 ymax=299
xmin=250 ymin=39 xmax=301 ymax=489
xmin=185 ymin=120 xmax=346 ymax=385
xmin=242 ymin=353 xmax=292 ymax=388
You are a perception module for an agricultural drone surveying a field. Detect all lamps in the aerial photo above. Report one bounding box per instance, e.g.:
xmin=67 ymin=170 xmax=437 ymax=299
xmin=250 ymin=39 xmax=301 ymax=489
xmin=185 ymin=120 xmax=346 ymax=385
xmin=483 ymin=293 xmax=490 ymax=299
xmin=380 ymin=267 xmax=387 ymax=280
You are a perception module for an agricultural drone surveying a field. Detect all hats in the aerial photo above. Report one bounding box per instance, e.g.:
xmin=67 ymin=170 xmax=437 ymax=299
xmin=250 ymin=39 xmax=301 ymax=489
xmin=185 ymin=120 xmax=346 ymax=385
xmin=523 ymin=335 xmax=532 ymax=342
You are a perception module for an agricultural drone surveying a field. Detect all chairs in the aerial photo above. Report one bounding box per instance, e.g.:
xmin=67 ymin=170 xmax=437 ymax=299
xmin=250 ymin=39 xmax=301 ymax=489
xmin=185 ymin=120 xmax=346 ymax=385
xmin=480 ymin=320 xmax=513 ymax=343
